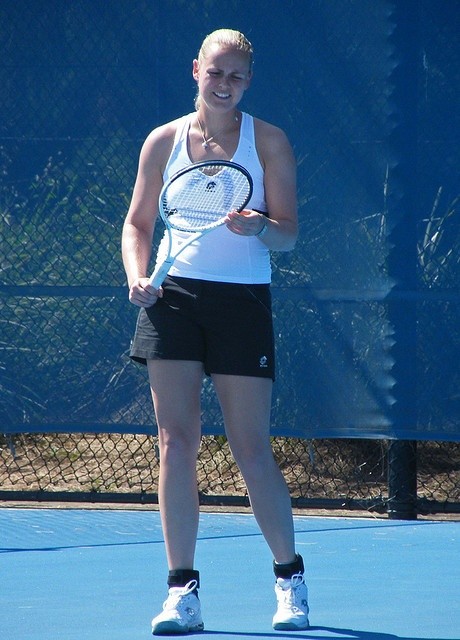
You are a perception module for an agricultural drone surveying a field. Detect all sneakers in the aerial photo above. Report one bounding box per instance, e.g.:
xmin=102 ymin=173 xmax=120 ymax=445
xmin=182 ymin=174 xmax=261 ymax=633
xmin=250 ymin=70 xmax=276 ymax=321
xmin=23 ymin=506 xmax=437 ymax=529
xmin=272 ymin=570 xmax=309 ymax=631
xmin=151 ymin=579 xmax=204 ymax=635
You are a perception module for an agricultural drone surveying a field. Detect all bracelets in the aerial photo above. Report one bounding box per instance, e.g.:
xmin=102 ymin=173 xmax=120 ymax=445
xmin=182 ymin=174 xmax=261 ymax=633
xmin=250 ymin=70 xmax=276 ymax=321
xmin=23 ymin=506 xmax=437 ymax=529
xmin=255 ymin=213 xmax=269 ymax=238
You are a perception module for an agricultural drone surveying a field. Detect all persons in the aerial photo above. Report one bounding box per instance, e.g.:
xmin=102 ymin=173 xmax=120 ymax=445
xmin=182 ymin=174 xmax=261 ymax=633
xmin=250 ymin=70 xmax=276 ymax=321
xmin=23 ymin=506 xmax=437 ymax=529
xmin=121 ymin=29 xmax=307 ymax=633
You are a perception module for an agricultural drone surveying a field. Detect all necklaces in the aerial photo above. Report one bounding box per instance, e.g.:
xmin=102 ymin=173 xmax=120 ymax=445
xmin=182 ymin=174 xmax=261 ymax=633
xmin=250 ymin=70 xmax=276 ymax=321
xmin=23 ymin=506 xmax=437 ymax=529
xmin=194 ymin=110 xmax=238 ymax=149
